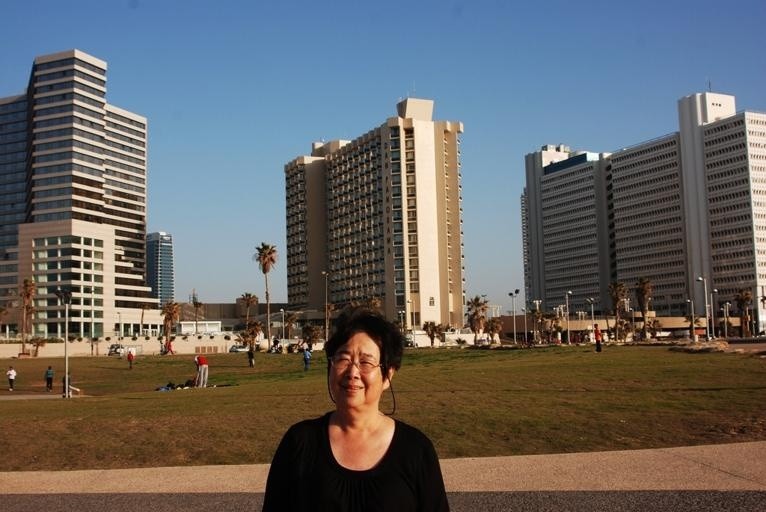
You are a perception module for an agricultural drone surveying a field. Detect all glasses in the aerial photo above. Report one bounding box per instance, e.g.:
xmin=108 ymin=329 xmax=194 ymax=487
xmin=330 ymin=358 xmax=380 ymax=372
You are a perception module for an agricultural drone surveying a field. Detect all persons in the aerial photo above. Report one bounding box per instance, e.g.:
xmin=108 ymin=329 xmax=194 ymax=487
xmin=163 ymin=342 xmax=168 ymax=355
xmin=5 ymin=366 xmax=17 ymax=391
xmin=163 ymin=342 xmax=175 ymax=355
xmin=303 ymin=348 xmax=312 ymax=370
xmin=44 ymin=365 xmax=55 ymax=392
xmin=594 ymin=323 xmax=604 ymax=352
xmin=194 ymin=355 xmax=208 ymax=387
xmin=127 ymin=351 xmax=134 ymax=369
xmin=273 ymin=338 xmax=280 ymax=349
xmin=247 ymin=349 xmax=255 ymax=368
xmin=62 ymin=370 xmax=72 ymax=398
xmin=262 ymin=305 xmax=452 ymax=511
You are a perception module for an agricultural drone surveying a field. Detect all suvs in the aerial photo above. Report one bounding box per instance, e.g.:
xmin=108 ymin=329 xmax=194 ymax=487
xmin=108 ymin=344 xmax=124 ymax=355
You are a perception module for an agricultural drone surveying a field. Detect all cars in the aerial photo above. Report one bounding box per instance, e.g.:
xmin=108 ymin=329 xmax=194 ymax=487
xmin=229 ymin=344 xmax=248 ymax=351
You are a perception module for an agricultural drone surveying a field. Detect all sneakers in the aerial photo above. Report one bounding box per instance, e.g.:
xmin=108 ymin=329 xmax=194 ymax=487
xmin=47 ymin=390 xmax=52 ymax=391
xmin=250 ymin=366 xmax=255 ymax=368
xmin=9 ymin=388 xmax=14 ymax=391
xmin=198 ymin=385 xmax=206 ymax=388
xmin=62 ymin=395 xmax=70 ymax=398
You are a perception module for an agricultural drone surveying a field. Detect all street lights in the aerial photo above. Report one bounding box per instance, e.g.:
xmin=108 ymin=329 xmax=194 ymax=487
xmin=55 ymin=288 xmax=72 ymax=399
xmin=279 ymin=308 xmax=284 ymax=338
xmin=686 ymin=299 xmax=695 ymax=341
xmin=408 ymin=299 xmax=415 ymax=347
xmin=116 ymin=311 xmax=122 ymax=354
xmin=697 ymin=277 xmax=710 ymax=341
xmin=398 ymin=311 xmax=405 ymax=333
xmin=710 ymin=289 xmax=718 ymax=340
xmin=508 ymin=290 xmax=594 ymax=345
xmin=321 ymin=272 xmax=329 ymax=344
